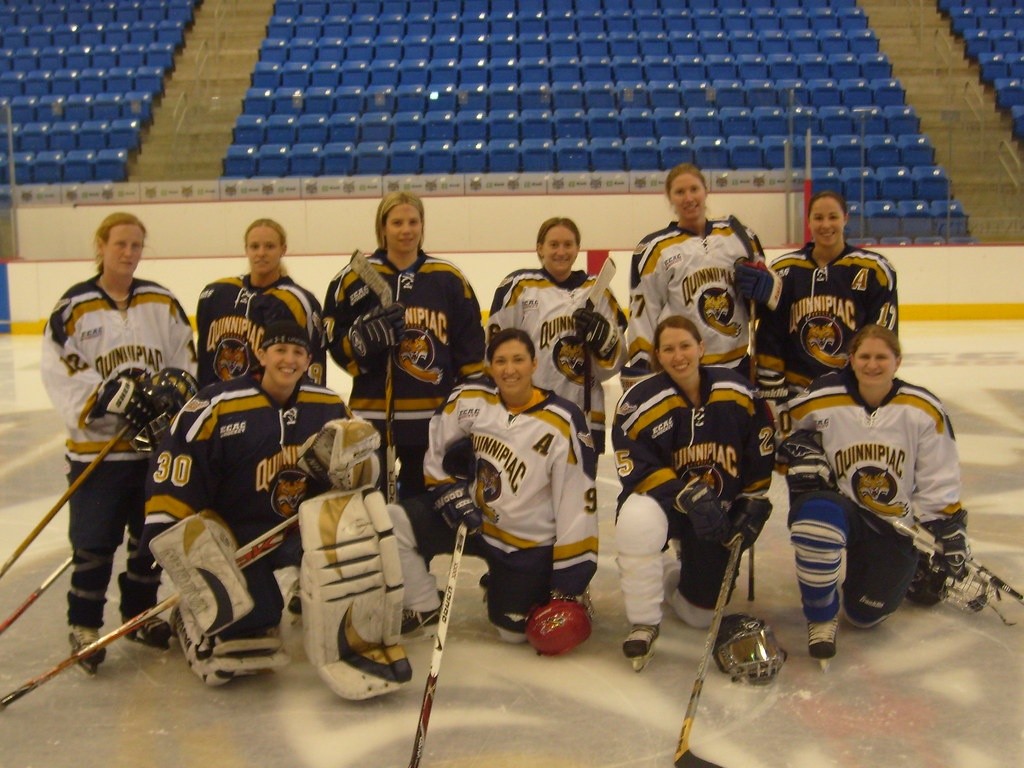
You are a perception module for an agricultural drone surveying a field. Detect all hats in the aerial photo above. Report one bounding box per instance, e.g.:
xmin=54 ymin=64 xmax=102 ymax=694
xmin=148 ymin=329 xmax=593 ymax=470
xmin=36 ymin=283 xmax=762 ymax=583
xmin=261 ymin=321 xmax=312 ymax=357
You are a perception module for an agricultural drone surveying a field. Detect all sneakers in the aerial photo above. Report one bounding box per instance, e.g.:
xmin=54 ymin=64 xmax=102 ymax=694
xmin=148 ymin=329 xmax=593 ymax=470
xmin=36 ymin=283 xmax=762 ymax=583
xmin=400 ymin=591 xmax=446 ymax=642
xmin=68 ymin=622 xmax=106 ymax=679
xmin=622 ymin=622 xmax=659 ymax=674
xmin=479 ymin=572 xmax=489 ymax=601
xmin=286 ymin=576 xmax=302 ymax=624
xmin=909 ymin=562 xmax=941 ymax=603
xmin=121 ymin=614 xmax=173 ymax=655
xmin=807 ymin=614 xmax=838 ymax=674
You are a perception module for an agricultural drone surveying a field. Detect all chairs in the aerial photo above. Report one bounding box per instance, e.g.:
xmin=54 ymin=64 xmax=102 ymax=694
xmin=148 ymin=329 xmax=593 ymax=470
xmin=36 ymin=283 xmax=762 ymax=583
xmin=0 ymin=0 xmax=200 ymax=187
xmin=220 ymin=0 xmax=1024 ymax=250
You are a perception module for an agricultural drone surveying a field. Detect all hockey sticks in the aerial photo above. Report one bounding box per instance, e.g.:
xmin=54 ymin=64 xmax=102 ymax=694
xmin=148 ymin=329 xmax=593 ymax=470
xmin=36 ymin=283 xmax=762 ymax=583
xmin=582 ymin=257 xmax=618 ymax=434
xmin=410 ymin=522 xmax=468 ymax=767
xmin=347 ymin=248 xmax=398 ymax=507
xmin=1 ymin=556 xmax=73 ymax=635
xmin=1 ymin=419 xmax=134 ymax=578
xmin=1 ymin=513 xmax=300 ymax=710
xmin=827 ymin=479 xmax=1024 ymax=604
xmin=672 ymin=537 xmax=744 ymax=768
xmin=726 ymin=213 xmax=757 ymax=389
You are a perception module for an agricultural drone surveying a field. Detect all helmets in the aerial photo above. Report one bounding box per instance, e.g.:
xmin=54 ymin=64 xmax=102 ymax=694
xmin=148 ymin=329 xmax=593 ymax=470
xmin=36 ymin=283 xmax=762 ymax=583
xmin=710 ymin=613 xmax=784 ymax=688
xmin=525 ymin=590 xmax=594 ymax=657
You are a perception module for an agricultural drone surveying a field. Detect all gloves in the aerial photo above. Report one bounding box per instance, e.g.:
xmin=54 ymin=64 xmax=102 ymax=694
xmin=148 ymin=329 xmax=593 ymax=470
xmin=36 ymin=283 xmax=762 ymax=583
xmin=921 ymin=506 xmax=969 ymax=583
xmin=720 ymin=494 xmax=773 ymax=554
xmin=572 ymin=308 xmax=618 ymax=359
xmin=681 ymin=483 xmax=731 ymax=544
xmin=347 ymin=302 xmax=406 ymax=363
xmin=427 ymin=484 xmax=482 ymax=537
xmin=777 ymin=429 xmax=837 ymax=493
xmin=755 ymin=371 xmax=797 ymax=403
xmin=733 ymin=260 xmax=786 ymax=310
xmin=96 ymin=379 xmax=158 ymax=430
xmin=131 ymin=366 xmax=198 ymax=455
xmin=619 ymin=368 xmax=658 ymax=393
xmin=468 ymin=374 xmax=492 ymax=388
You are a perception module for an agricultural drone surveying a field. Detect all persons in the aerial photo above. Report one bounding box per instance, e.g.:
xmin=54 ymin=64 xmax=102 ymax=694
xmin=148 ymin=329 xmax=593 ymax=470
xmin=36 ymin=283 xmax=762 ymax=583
xmin=612 ymin=316 xmax=776 ymax=673
xmin=774 ymin=324 xmax=969 ymax=672
xmin=323 ymin=191 xmax=489 ymax=517
xmin=40 ymin=212 xmax=197 ymax=675
xmin=486 ymin=217 xmax=629 ymax=483
xmin=754 ymin=190 xmax=898 ymax=470
xmin=134 ymin=321 xmax=380 ymax=686
xmin=197 ymin=219 xmax=326 ymax=393
xmin=627 ymin=162 xmax=764 ymax=559
xmin=383 ymin=326 xmax=601 ymax=646
